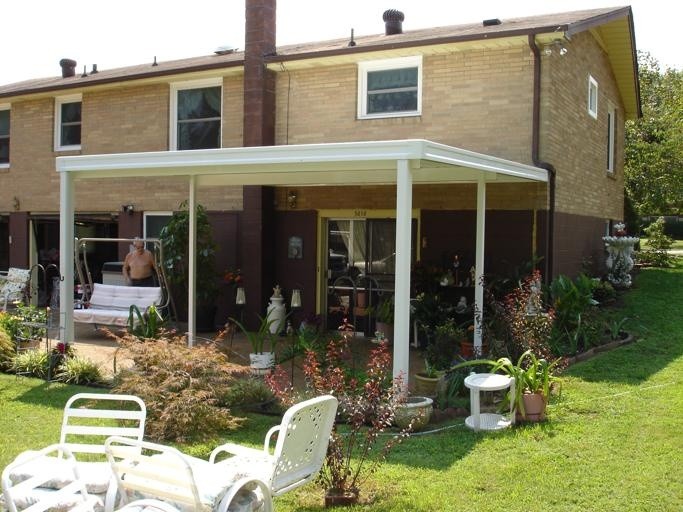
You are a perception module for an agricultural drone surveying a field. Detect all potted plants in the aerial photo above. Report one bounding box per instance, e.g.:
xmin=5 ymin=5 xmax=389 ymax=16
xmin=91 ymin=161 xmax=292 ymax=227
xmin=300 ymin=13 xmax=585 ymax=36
xmin=369 ymin=297 xmax=563 ymax=423
xmin=227 ymin=308 xmax=294 ymax=376
xmin=156 ymin=199 xmax=223 ymax=333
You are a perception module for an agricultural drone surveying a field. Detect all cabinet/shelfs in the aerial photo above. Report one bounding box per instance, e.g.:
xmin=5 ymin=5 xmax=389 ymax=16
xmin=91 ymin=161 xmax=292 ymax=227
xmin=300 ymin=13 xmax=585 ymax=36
xmin=14 ymin=310 xmax=66 ymax=388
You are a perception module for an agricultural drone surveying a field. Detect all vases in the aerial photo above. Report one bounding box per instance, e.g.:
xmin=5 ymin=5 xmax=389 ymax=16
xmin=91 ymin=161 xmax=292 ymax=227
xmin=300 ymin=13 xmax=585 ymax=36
xmin=390 ymin=397 xmax=434 ymax=430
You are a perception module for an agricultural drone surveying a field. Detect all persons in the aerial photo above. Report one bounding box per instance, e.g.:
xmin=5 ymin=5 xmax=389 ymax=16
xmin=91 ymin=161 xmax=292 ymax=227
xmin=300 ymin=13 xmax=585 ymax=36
xmin=121 ymin=236 xmax=163 ymax=287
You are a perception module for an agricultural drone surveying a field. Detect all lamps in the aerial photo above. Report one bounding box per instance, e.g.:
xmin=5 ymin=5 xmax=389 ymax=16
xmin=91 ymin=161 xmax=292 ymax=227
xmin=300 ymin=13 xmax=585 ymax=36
xmin=289 ymin=288 xmax=302 ymax=388
xmin=234 ymin=285 xmax=246 ymax=333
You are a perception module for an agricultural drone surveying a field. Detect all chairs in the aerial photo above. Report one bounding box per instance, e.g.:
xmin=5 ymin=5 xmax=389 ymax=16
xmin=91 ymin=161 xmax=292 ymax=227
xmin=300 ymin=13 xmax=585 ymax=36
xmin=0 ymin=267 xmax=33 ymax=310
xmin=7 ymin=393 xmax=147 ymax=510
xmin=208 ymin=394 xmax=337 ymax=499
xmin=2 ymin=444 xmax=177 ymax=511
xmin=103 ymin=436 xmax=272 ymax=512
